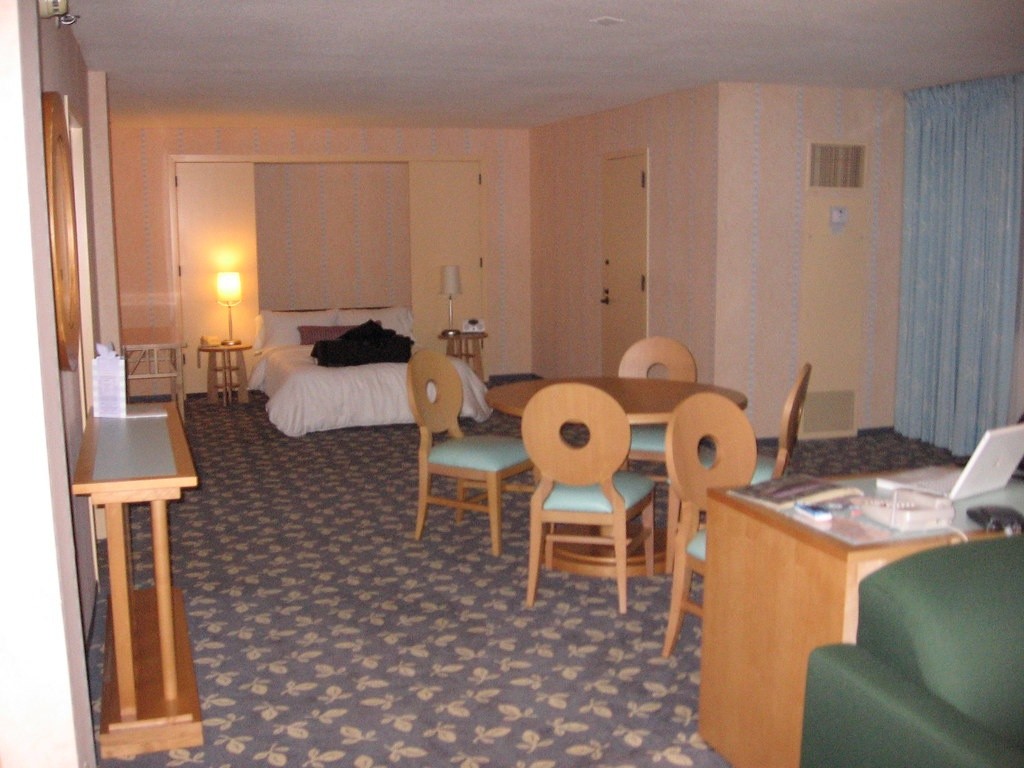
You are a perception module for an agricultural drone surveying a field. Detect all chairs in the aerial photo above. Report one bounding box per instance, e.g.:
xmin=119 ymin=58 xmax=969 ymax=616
xmin=618 ymin=336 xmax=697 ymax=482
xmin=520 ymin=382 xmax=655 ymax=614
xmin=406 ymin=349 xmax=530 ymax=558
xmin=123 ymin=344 xmax=185 ymax=418
xmin=661 ymin=364 xmax=812 ymax=660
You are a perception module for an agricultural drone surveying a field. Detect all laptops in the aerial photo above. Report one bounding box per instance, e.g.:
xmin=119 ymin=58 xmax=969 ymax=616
xmin=876 ymin=424 xmax=1024 ymax=502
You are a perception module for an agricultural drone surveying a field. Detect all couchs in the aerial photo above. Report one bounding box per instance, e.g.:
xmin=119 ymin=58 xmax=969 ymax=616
xmin=798 ymin=535 xmax=1024 ymax=768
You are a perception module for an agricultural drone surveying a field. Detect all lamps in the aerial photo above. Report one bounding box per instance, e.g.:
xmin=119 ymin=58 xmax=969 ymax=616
xmin=440 ymin=265 xmax=461 ymax=335
xmin=216 ymin=271 xmax=241 ymax=345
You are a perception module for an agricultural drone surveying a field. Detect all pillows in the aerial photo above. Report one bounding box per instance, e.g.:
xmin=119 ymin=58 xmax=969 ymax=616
xmin=261 ymin=307 xmax=417 ymax=346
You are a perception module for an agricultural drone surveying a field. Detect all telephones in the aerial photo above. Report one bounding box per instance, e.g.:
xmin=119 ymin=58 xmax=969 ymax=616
xmin=861 ymin=488 xmax=957 ymax=533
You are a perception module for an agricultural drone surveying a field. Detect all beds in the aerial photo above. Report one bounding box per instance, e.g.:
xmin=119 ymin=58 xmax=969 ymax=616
xmin=256 ymin=305 xmax=487 ymax=437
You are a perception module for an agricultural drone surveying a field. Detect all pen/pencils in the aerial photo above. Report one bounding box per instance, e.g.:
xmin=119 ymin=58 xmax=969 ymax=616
xmin=816 ymin=503 xmax=853 ymax=510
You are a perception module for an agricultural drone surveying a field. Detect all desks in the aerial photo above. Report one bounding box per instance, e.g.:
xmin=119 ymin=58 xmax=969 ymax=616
xmin=437 ymin=332 xmax=488 ymax=380
xmin=199 ymin=344 xmax=253 ymax=406
xmin=485 ymin=377 xmax=747 ymax=577
xmin=71 ymin=401 xmax=203 ymax=759
xmin=700 ymin=464 xmax=1024 ymax=768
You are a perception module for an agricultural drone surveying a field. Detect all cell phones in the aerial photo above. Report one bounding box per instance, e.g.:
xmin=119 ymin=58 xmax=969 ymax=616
xmin=793 ymin=504 xmax=833 ymax=521
